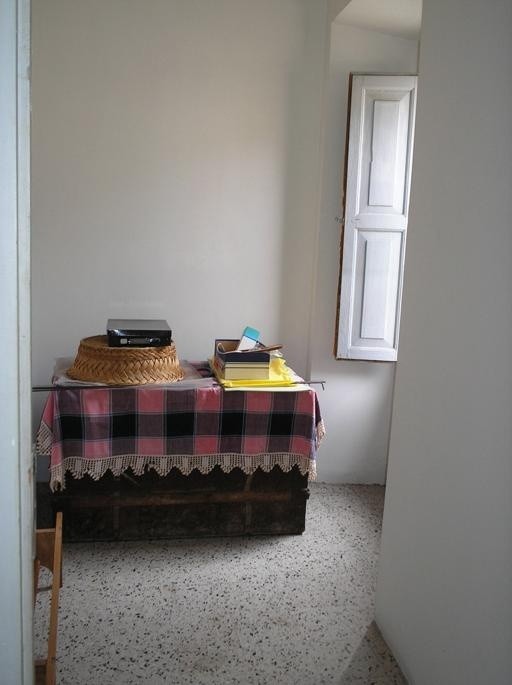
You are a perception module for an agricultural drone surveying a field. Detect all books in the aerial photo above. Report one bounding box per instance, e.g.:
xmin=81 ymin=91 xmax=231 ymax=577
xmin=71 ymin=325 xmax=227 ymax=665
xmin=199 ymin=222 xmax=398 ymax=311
xmin=214 ymin=352 xmax=270 ymax=381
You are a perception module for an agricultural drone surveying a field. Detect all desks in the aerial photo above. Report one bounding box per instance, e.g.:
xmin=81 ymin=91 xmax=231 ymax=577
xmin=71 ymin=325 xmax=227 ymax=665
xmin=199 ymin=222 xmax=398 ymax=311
xmin=36 ymin=362 xmax=327 ymax=542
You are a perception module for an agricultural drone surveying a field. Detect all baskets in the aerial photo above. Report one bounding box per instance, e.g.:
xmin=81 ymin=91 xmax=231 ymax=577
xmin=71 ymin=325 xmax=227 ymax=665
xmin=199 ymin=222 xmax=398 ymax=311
xmin=67 ymin=334 xmax=187 ymax=388
